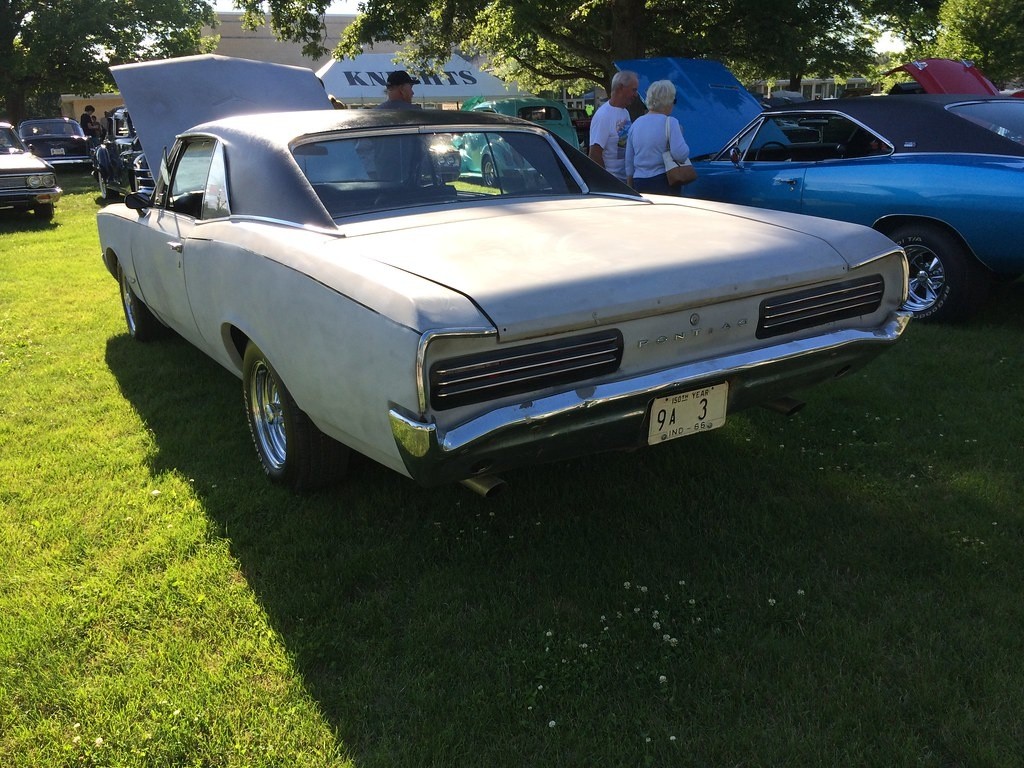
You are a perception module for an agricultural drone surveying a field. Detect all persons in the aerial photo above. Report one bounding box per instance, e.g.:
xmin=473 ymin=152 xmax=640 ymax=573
xmin=587 ymin=68 xmax=639 ymax=185
xmin=370 ymin=69 xmax=427 ymax=181
xmin=81 ymin=105 xmax=110 ymax=144
xmin=625 ymin=80 xmax=691 ymax=197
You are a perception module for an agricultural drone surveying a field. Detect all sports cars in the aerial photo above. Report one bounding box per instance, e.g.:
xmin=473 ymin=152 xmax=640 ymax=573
xmin=866 ymin=58 xmax=1024 ymax=157
xmin=96 ymin=52 xmax=914 ymax=499
xmin=610 ymin=56 xmax=1024 ymax=325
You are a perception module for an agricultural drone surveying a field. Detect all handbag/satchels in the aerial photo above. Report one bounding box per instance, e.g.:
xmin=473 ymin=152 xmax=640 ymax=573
xmin=662 ymin=116 xmax=697 ymax=186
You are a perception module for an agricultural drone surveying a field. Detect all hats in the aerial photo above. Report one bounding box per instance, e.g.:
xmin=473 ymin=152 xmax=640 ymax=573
xmin=386 ymin=70 xmax=420 ymax=87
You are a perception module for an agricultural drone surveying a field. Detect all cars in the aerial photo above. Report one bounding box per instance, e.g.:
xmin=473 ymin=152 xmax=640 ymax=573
xmin=313 ymin=51 xmax=890 ymax=184
xmin=0 ymin=121 xmax=63 ymax=222
xmin=450 ymin=94 xmax=579 ymax=192
xmin=90 ymin=105 xmax=156 ymax=201
xmin=17 ymin=116 xmax=93 ymax=168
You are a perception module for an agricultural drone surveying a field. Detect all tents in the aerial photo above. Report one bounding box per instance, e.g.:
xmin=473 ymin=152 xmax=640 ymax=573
xmin=316 ymin=50 xmax=536 ymax=109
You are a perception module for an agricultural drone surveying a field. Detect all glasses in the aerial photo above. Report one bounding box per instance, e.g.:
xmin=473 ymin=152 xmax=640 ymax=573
xmin=671 ymin=98 xmax=677 ymax=105
